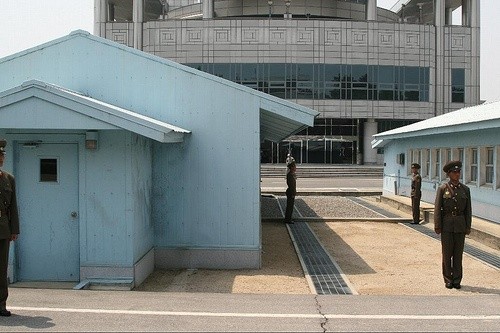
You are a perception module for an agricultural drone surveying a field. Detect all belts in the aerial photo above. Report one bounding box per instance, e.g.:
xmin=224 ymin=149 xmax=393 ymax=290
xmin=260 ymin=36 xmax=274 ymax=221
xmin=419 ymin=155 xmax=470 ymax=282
xmin=412 ymin=189 xmax=420 ymax=191
xmin=444 ymin=211 xmax=465 ymax=216
xmin=289 ymin=187 xmax=296 ymax=189
xmin=0 ymin=210 xmax=9 ymax=218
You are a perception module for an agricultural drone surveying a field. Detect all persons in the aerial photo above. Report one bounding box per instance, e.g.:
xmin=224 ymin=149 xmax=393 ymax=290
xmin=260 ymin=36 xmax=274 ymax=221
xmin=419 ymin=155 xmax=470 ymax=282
xmin=434 ymin=161 xmax=473 ymax=290
xmin=408 ymin=163 xmax=425 ymax=227
xmin=285 ymin=153 xmax=297 ymax=179
xmin=0 ymin=139 xmax=21 ymax=316
xmin=284 ymin=162 xmax=300 ymax=226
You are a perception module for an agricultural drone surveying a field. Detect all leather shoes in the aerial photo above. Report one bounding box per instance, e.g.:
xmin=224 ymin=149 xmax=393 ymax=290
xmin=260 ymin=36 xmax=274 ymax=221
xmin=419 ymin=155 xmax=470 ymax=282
xmin=285 ymin=221 xmax=294 ymax=224
xmin=0 ymin=310 xmax=11 ymax=316
xmin=454 ymin=283 xmax=461 ymax=289
xmin=410 ymin=221 xmax=419 ymax=224
xmin=446 ymin=282 xmax=453 ymax=289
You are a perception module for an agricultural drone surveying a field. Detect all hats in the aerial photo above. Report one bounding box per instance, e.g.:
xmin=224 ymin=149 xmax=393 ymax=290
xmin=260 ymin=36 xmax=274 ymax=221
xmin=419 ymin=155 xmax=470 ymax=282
xmin=411 ymin=164 xmax=421 ymax=169
xmin=0 ymin=140 xmax=7 ymax=155
xmin=287 ymin=162 xmax=296 ymax=168
xmin=443 ymin=161 xmax=462 ymax=173
